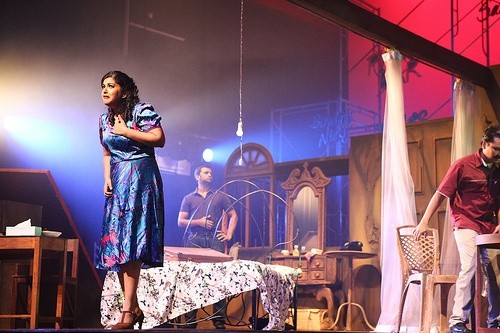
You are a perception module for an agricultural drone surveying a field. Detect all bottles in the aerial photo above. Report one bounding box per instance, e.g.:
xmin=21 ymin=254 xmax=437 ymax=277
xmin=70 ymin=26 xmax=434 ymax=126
xmin=292 ymin=246 xmax=300 ymax=256
xmin=300 ymin=246 xmax=306 ymax=252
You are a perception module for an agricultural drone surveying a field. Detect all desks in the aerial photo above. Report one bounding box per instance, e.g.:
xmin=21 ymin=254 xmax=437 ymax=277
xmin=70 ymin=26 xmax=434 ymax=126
xmin=323 ymin=250 xmax=378 ymax=331
xmin=0 ymin=237 xmax=68 ymax=329
xmin=474 ymin=234 xmax=500 ymax=333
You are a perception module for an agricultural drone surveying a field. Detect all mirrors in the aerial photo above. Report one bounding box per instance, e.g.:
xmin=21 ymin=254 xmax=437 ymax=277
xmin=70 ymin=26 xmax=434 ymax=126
xmin=281 ymin=162 xmax=331 ymax=254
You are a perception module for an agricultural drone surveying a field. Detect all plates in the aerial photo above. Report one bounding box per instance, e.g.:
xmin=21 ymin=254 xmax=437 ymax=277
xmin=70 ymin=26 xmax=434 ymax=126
xmin=289 ymin=252 xmax=307 ymax=255
xmin=41 ymin=231 xmax=62 ymax=237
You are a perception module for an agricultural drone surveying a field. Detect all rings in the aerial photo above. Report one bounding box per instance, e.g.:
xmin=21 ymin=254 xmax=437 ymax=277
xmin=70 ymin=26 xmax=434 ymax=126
xmin=416 ymin=233 xmax=418 ymax=236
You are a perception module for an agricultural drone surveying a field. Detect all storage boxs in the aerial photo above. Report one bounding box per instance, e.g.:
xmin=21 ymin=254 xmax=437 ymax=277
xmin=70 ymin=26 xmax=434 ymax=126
xmin=285 ymin=308 xmax=337 ymax=331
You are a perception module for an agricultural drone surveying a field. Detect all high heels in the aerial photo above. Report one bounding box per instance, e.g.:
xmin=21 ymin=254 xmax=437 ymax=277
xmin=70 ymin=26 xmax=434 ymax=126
xmin=110 ymin=309 xmax=144 ymax=330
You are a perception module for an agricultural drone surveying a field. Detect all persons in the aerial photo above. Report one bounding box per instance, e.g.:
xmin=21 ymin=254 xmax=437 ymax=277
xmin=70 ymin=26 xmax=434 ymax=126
xmin=95 ymin=70 xmax=166 ymax=330
xmin=177 ymin=165 xmax=238 ymax=329
xmin=412 ymin=123 xmax=500 ymax=333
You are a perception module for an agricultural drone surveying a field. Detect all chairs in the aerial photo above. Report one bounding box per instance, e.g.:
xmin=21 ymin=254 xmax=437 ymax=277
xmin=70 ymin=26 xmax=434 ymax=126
xmin=9 ymin=238 xmax=80 ymax=330
xmin=396 ymin=225 xmax=476 ymax=333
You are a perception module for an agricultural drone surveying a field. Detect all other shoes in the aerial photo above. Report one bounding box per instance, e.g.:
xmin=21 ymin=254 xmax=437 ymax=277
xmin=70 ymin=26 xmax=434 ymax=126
xmin=182 ymin=326 xmax=197 ymax=329
xmin=215 ymin=323 xmax=225 ymax=329
xmin=450 ymin=323 xmax=471 ymax=333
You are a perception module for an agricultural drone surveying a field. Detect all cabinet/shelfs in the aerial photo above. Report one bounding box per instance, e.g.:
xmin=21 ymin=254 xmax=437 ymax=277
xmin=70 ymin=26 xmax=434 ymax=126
xmin=266 ymin=255 xmax=344 ymax=331
